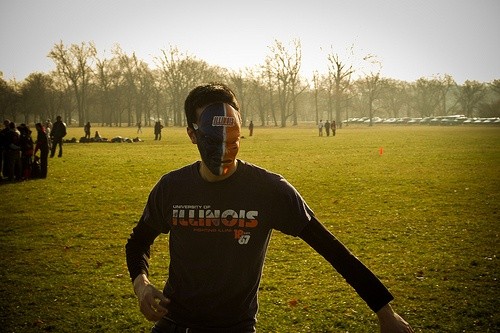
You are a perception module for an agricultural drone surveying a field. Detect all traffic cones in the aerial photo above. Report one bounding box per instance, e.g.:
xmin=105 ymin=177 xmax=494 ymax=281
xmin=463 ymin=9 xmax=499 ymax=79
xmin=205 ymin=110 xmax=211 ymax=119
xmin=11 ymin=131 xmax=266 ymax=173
xmin=379 ymin=146 xmax=383 ymax=155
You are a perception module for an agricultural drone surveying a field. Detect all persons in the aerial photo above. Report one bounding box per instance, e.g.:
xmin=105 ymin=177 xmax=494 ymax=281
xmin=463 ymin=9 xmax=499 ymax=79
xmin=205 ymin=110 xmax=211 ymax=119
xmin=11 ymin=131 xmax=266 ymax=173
xmin=126 ymin=81 xmax=415 ymax=333
xmin=158 ymin=121 xmax=163 ymax=140
xmin=49 ymin=115 xmax=67 ymax=158
xmin=248 ymin=121 xmax=254 ymax=136
xmin=331 ymin=121 xmax=336 ymax=136
xmin=153 ymin=122 xmax=160 ymax=139
xmin=318 ymin=120 xmax=323 ymax=136
xmin=0 ymin=116 xmax=52 ymax=184
xmin=325 ymin=120 xmax=331 ymax=136
xmin=84 ymin=122 xmax=91 ymax=138
xmin=94 ymin=130 xmax=102 ymax=138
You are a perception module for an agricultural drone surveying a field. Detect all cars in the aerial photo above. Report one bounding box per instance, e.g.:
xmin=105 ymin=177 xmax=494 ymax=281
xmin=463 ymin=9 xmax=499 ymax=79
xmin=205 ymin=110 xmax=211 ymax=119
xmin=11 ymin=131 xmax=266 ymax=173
xmin=343 ymin=115 xmax=500 ymax=125
